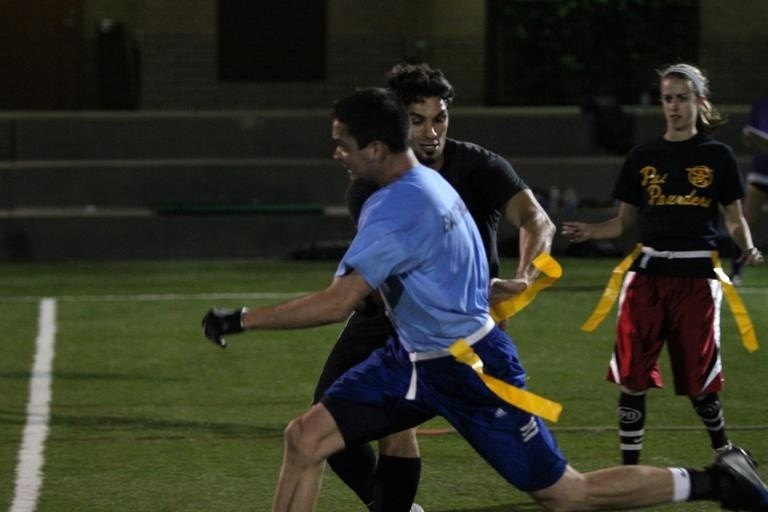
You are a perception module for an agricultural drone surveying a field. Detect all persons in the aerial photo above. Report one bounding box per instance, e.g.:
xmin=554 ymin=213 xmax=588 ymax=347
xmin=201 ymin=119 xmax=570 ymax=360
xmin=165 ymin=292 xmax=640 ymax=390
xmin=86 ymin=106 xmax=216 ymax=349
xmin=560 ymin=61 xmax=766 ymax=471
xmin=726 ymin=71 xmax=768 ymax=288
xmin=199 ymin=85 xmax=767 ymax=512
xmin=309 ymin=59 xmax=560 ymax=512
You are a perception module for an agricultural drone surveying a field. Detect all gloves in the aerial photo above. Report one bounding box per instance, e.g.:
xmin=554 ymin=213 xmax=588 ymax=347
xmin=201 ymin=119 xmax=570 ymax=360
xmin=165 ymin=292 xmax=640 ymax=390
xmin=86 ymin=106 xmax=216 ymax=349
xmin=200 ymin=306 xmax=245 ymax=350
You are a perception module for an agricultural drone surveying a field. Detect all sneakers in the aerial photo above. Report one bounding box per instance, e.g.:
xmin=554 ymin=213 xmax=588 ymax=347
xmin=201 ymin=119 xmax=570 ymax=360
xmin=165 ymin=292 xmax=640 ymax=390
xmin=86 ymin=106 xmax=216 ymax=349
xmin=712 ymin=442 xmax=768 ymax=512
